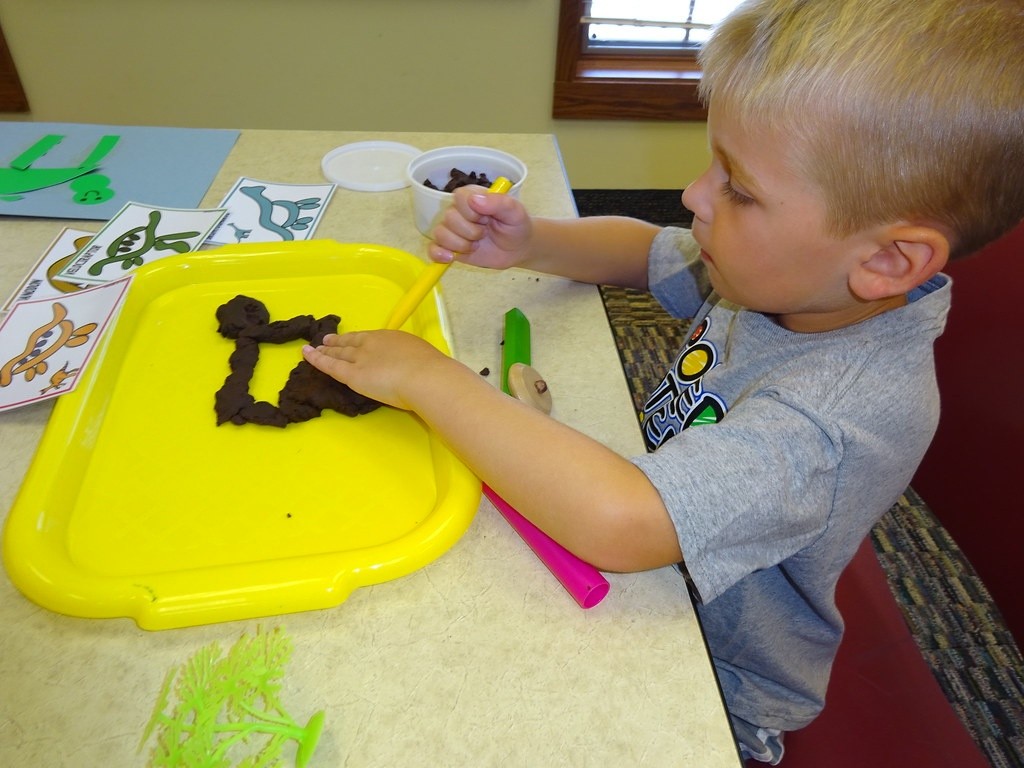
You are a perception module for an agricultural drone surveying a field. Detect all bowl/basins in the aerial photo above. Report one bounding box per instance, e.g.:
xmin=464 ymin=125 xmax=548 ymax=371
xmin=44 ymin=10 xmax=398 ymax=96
xmin=405 ymin=145 xmax=528 ymax=239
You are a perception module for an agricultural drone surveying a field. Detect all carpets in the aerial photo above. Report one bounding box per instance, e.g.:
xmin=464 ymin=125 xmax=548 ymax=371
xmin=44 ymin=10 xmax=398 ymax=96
xmin=776 ymin=536 xmax=986 ymax=768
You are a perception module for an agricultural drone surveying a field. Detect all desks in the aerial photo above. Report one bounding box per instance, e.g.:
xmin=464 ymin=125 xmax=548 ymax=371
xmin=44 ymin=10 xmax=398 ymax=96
xmin=0 ymin=122 xmax=742 ymax=768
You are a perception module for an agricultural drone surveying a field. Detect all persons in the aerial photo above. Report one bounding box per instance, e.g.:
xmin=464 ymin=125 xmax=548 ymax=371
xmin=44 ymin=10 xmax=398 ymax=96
xmin=301 ymin=0 xmax=1024 ymax=763
